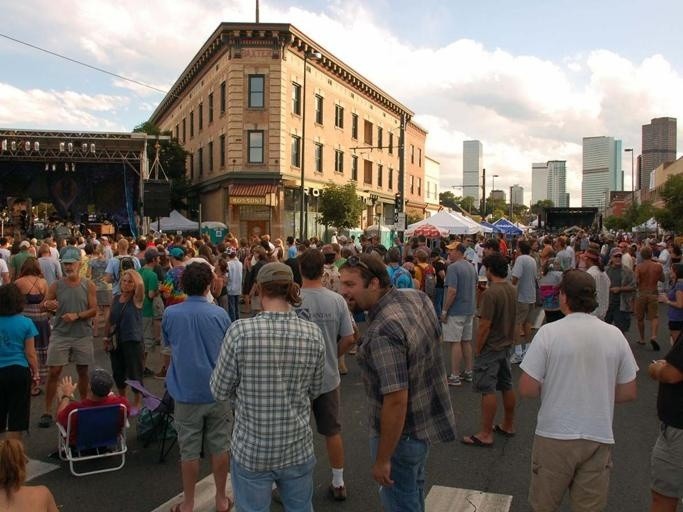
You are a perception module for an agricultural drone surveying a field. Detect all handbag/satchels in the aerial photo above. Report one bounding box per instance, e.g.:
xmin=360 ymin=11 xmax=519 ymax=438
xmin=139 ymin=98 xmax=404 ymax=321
xmin=103 ymin=322 xmax=116 ymax=352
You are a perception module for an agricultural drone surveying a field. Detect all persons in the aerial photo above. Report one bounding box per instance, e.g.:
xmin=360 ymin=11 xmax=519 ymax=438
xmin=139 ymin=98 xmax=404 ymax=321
xmin=338 ymin=252 xmax=459 ymax=511
xmin=208 ymin=261 xmax=327 ymax=511
xmin=644 ymin=322 xmax=682 ymax=511
xmin=159 ymin=261 xmax=234 ymax=511
xmin=516 ymin=267 xmax=642 ymax=511
xmin=286 ymin=247 xmax=357 ymax=501
xmin=1 ymin=207 xmax=683 ymax=446
xmin=0 ymin=437 xmax=60 ymax=512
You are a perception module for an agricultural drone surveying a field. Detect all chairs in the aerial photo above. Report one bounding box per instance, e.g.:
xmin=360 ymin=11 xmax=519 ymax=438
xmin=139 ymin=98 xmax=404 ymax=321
xmin=56 ymin=404 xmax=129 ymax=477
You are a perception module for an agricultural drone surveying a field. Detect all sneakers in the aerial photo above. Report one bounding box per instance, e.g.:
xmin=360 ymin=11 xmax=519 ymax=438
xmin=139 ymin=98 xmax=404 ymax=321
xmin=129 ymin=406 xmax=140 ymax=417
xmin=447 ymin=369 xmax=472 ymax=386
xmin=329 ymin=480 xmax=347 ymax=501
xmin=272 ymin=488 xmax=281 ymax=502
xmin=338 ymin=344 xmax=357 ymax=374
xmin=510 ymin=351 xmax=526 ymax=364
xmin=143 ymin=337 xmax=166 ymax=380
xmin=39 ymin=414 xmax=52 ymax=428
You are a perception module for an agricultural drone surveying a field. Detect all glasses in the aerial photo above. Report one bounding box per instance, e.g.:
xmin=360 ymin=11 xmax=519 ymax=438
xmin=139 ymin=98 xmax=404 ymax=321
xmin=345 ymin=255 xmax=368 ymax=269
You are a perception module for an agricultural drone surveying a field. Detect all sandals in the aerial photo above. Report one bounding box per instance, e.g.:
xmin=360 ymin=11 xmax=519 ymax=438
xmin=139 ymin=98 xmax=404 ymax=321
xmin=216 ymin=496 xmax=234 ymax=512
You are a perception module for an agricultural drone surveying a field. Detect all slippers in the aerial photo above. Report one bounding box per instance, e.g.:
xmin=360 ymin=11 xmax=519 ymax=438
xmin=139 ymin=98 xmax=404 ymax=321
xmin=636 ymin=337 xmax=660 ymax=351
xmin=493 ymin=423 xmax=515 ymax=437
xmin=171 ymin=501 xmax=193 ymax=512
xmin=461 ymin=434 xmax=493 ymax=447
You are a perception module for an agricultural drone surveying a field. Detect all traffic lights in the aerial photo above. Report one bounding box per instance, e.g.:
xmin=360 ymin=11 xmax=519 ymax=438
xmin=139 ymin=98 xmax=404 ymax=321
xmin=395 ymin=192 xmax=402 ymax=213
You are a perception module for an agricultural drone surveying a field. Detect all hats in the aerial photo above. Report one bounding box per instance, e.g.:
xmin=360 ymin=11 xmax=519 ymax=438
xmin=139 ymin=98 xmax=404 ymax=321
xmin=605 ymin=233 xmax=622 ymax=256
xmin=145 ymin=246 xmax=164 ymax=259
xmin=445 ymin=242 xmax=466 ymax=252
xmin=649 ymin=238 xmax=666 ymax=248
xmin=93 ymin=236 xmax=108 ymax=245
xmin=480 ymin=238 xmax=499 ymax=248
xmin=19 ymin=238 xmax=37 ymax=249
xmin=261 ymin=235 xmax=270 ymax=240
xmin=257 ymin=262 xmax=294 ymax=284
xmin=60 ymin=249 xmax=80 ymax=264
xmin=224 ymin=245 xmax=236 ymax=255
xmin=167 ymin=248 xmax=185 ymax=259
xmin=90 ymin=370 xmax=113 ymax=390
xmin=322 ymin=235 xmax=347 ymax=254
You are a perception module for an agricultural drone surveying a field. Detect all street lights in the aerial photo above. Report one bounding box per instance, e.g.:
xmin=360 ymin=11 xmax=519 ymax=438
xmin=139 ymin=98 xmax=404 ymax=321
xmin=624 ymin=148 xmax=633 ymax=195
xmin=299 ymin=51 xmax=322 ymax=246
xmin=491 ymin=174 xmax=498 ymax=213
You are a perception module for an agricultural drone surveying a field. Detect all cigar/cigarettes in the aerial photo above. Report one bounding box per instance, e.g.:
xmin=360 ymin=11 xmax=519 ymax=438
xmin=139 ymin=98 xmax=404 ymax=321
xmin=378 ymin=484 xmax=385 ymax=491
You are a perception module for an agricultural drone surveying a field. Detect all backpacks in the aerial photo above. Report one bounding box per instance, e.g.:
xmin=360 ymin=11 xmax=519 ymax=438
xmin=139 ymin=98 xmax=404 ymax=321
xmin=388 ymin=265 xmax=411 ymax=289
xmin=114 ymin=255 xmax=134 ymax=281
xmin=415 ymin=264 xmax=435 ymax=296
